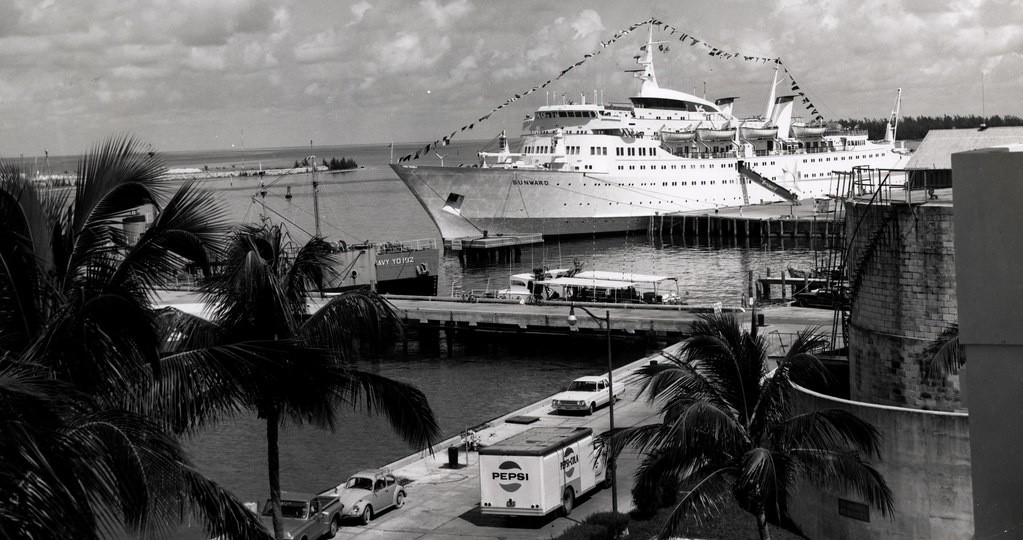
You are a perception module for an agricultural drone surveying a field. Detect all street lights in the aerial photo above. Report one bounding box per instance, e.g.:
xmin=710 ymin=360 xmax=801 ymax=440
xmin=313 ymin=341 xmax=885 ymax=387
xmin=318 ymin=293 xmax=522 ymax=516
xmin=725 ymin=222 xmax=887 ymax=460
xmin=567 ymin=300 xmax=620 ymax=540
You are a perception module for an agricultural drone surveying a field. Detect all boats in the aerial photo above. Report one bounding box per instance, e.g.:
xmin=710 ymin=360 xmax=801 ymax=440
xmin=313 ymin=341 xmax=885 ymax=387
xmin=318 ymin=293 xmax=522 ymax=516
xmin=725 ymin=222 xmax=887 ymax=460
xmin=739 ymin=126 xmax=778 ymax=139
xmin=70 ymin=139 xmax=441 ymax=298
xmin=790 ymin=125 xmax=826 ymax=137
xmin=493 ymin=267 xmax=691 ymax=305
xmin=661 ymin=131 xmax=696 ymax=143
xmin=696 ymin=127 xmax=737 ymax=141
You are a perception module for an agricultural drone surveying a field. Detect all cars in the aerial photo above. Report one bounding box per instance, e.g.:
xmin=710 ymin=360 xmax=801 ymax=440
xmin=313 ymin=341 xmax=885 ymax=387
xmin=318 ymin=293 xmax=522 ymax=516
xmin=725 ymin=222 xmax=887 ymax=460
xmin=257 ymin=491 xmax=344 ymax=540
xmin=551 ymin=375 xmax=625 ymax=415
xmin=337 ymin=469 xmax=407 ymax=525
xmin=792 ymin=286 xmax=852 ymax=311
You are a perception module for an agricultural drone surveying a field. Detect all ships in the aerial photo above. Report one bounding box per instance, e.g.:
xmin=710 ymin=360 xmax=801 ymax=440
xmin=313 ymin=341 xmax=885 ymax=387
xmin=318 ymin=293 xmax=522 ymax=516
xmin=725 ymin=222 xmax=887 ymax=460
xmin=386 ymin=15 xmax=914 ymax=255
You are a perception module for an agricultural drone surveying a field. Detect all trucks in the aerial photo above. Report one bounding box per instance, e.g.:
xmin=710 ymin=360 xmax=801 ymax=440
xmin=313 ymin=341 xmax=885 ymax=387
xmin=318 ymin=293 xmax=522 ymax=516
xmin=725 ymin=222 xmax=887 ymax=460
xmin=477 ymin=424 xmax=618 ymax=518
